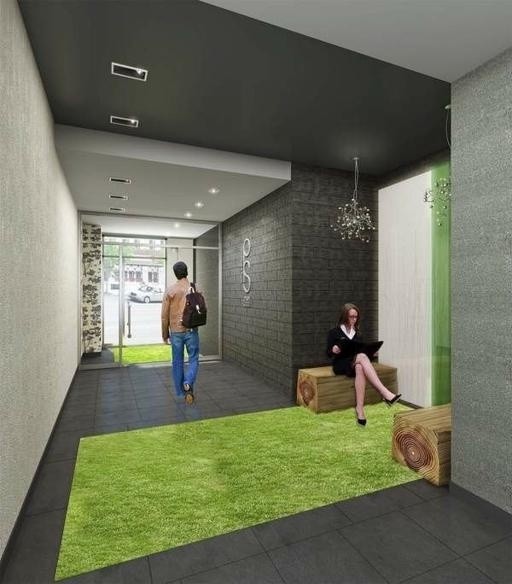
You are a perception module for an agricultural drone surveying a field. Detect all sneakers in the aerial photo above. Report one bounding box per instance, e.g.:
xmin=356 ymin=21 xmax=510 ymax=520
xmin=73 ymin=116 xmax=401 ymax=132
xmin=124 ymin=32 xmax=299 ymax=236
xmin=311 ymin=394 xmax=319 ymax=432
xmin=182 ymin=383 xmax=194 ymax=406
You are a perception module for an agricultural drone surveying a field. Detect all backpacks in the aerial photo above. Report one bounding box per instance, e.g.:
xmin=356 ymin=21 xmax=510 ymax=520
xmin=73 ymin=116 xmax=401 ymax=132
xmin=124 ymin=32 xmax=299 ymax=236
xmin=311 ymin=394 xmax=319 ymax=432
xmin=182 ymin=282 xmax=207 ymax=328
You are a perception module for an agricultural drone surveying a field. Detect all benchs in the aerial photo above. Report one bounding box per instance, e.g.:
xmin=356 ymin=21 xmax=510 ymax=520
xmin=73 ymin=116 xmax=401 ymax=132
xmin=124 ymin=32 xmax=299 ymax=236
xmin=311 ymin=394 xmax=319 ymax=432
xmin=295 ymin=362 xmax=399 ymax=413
xmin=390 ymin=401 xmax=455 ymax=484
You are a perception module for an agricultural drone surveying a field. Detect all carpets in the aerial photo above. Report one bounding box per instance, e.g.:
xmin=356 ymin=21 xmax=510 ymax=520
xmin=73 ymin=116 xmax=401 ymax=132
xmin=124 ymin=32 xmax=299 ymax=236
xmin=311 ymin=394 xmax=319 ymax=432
xmin=53 ymin=396 xmax=444 ymax=582
xmin=106 ymin=342 xmax=206 ymax=367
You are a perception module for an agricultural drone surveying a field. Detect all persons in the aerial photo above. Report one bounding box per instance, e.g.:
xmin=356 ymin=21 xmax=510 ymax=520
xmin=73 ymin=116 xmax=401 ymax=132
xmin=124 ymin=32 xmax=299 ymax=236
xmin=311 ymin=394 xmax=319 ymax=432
xmin=326 ymin=302 xmax=402 ymax=426
xmin=161 ymin=261 xmax=200 ymax=407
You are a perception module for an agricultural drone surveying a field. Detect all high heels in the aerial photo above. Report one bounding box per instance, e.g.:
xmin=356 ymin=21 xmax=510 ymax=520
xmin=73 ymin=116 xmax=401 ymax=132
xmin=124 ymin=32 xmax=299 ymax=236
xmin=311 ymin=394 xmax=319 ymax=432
xmin=355 ymin=408 xmax=366 ymax=426
xmin=385 ymin=394 xmax=401 ymax=407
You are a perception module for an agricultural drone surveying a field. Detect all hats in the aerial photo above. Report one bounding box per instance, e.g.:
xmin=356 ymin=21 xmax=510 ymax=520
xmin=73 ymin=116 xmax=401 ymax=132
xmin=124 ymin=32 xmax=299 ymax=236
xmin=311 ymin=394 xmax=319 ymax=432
xmin=173 ymin=261 xmax=187 ymax=279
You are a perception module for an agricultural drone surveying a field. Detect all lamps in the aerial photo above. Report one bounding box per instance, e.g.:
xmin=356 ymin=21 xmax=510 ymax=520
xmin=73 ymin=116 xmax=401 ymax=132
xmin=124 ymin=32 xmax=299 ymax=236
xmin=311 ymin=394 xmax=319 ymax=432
xmin=331 ymin=157 xmax=377 ymax=245
xmin=421 ymin=101 xmax=451 ymax=226
